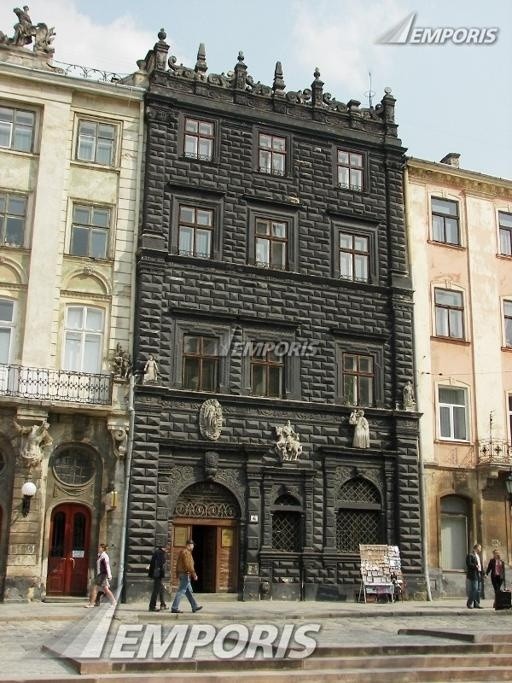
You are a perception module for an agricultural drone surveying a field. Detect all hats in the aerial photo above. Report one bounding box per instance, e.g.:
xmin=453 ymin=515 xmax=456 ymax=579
xmin=186 ymin=540 xmax=196 ymax=546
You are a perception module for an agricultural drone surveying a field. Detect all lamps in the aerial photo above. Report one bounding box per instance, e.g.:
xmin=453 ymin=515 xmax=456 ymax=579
xmin=21 ymin=481 xmax=37 ymax=515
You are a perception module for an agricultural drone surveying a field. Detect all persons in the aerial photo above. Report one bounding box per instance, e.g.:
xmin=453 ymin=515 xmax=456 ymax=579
xmin=144 ymin=355 xmax=159 ymax=380
xmin=283 ymin=420 xmax=299 ymax=451
xmin=148 ymin=540 xmax=203 ymax=613
xmin=350 ymin=409 xmax=370 ymax=448
xmin=466 ymin=544 xmax=507 ymax=609
xmin=403 ymin=381 xmax=415 ymax=406
xmin=84 ymin=544 xmax=117 ymax=608
xmin=206 ymin=401 xmax=217 ymax=426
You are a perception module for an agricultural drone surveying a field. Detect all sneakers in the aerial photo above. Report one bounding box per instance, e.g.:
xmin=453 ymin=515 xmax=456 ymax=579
xmin=160 ymin=606 xmax=169 ymax=609
xmin=149 ymin=608 xmax=160 ymax=612
xmin=84 ymin=605 xmax=94 ymax=608
xmin=109 ymin=601 xmax=117 ymax=608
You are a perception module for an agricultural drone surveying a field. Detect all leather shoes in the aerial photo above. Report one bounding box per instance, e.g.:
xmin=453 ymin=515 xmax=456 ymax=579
xmin=171 ymin=611 xmax=183 ymax=613
xmin=192 ymin=606 xmax=202 ymax=613
xmin=467 ymin=603 xmax=483 ymax=609
